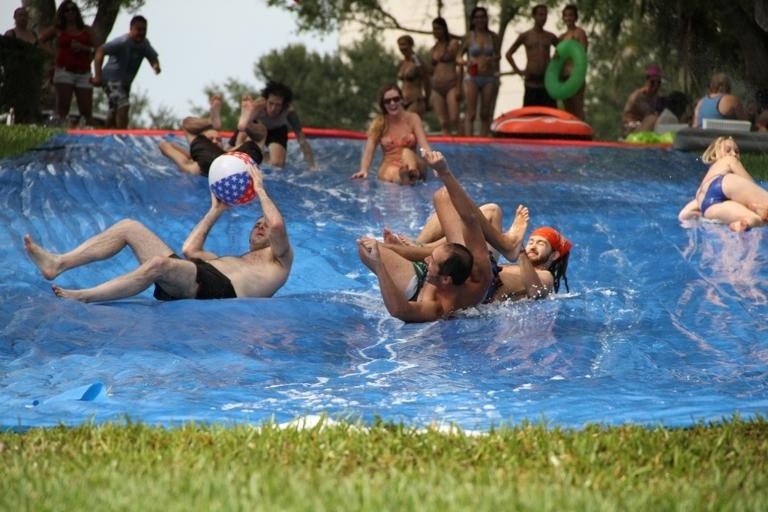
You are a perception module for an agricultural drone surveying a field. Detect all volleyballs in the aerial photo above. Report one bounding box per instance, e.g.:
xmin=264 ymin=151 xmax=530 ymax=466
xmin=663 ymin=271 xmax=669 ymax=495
xmin=208 ymin=152 xmax=259 ymax=205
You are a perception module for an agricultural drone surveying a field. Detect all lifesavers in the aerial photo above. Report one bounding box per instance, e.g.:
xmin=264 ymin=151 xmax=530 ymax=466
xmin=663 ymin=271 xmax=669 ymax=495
xmin=544 ymin=39 xmax=586 ymax=100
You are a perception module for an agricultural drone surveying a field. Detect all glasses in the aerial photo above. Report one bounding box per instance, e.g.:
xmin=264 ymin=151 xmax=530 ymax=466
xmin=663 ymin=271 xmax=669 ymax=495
xmin=382 ymin=97 xmax=404 ymax=105
